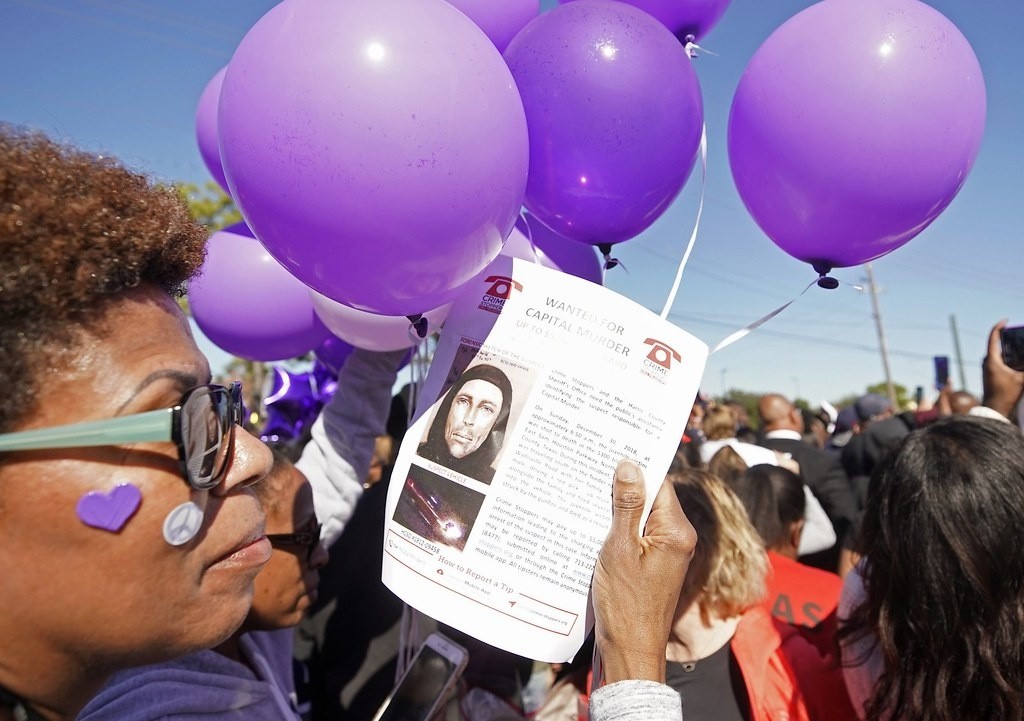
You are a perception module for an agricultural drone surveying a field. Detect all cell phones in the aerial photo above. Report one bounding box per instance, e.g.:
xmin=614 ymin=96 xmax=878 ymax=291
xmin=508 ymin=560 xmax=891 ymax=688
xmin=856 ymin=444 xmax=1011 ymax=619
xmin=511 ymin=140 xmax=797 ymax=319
xmin=917 ymin=387 xmax=922 ymax=405
xmin=374 ymin=630 xmax=470 ymax=721
xmin=934 ymin=357 xmax=948 ymax=391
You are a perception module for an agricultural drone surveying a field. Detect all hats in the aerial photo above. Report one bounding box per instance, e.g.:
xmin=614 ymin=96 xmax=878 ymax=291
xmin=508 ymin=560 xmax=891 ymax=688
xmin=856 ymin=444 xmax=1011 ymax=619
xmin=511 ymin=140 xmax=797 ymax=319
xmin=856 ymin=396 xmax=892 ymax=418
xmin=828 ymin=409 xmax=860 ymax=448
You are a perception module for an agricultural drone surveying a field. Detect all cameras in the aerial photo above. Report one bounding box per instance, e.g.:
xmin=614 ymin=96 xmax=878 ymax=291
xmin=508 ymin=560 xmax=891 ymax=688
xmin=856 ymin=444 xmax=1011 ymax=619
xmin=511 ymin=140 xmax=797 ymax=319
xmin=1000 ymin=325 xmax=1024 ymax=371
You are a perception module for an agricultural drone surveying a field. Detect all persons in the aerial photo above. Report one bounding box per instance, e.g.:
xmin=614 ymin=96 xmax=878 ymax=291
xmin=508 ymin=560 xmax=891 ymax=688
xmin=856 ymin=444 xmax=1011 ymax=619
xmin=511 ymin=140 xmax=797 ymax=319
xmin=417 ymin=363 xmax=513 ymax=485
xmin=77 ymin=316 xmax=1024 ymax=721
xmin=0 ymin=120 xmax=699 ymax=721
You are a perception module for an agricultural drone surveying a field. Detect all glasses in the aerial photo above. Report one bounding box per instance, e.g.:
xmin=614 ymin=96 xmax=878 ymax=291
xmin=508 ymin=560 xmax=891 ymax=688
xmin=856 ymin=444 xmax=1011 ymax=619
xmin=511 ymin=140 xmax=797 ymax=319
xmin=265 ymin=523 xmax=322 ymax=564
xmin=0 ymin=382 xmax=245 ymax=490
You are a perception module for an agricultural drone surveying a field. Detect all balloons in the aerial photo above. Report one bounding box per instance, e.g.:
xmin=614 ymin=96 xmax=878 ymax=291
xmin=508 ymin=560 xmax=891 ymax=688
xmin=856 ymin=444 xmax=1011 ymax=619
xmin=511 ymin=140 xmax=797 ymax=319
xmin=186 ymin=0 xmax=988 ymax=441
